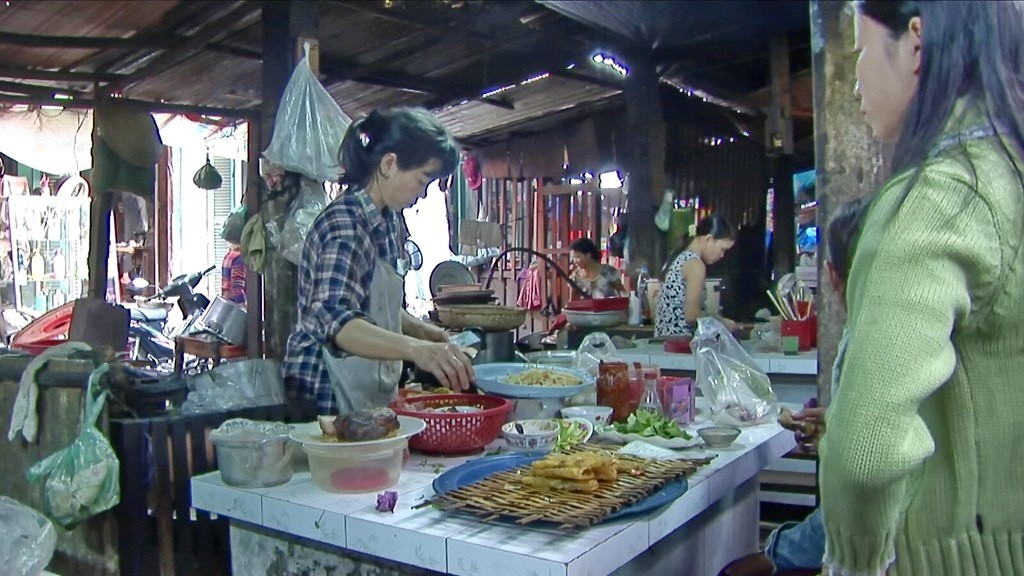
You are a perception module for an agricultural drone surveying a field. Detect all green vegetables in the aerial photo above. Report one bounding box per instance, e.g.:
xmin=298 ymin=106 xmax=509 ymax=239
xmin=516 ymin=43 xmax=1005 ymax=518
xmin=603 ymin=410 xmax=692 ymax=442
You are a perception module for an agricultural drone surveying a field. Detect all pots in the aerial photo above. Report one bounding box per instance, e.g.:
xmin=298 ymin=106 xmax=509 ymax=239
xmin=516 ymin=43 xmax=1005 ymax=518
xmin=646 ymin=278 xmax=726 ymax=323
xmin=445 ymin=326 xmax=514 ymax=366
xmin=198 ymin=291 xmax=263 ymax=346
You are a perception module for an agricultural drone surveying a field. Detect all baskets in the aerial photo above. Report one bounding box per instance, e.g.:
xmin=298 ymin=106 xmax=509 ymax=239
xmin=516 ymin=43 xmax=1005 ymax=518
xmin=437 ymin=304 xmax=529 ymax=331
xmin=389 ymin=394 xmax=515 ymax=455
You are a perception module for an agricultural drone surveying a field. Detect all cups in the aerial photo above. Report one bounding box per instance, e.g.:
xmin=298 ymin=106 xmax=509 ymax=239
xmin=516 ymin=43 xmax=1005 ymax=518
xmin=781 ymin=319 xmax=810 ymax=350
xmin=782 ymin=336 xmax=799 ymax=355
xmin=597 ymin=360 xmax=661 ymax=424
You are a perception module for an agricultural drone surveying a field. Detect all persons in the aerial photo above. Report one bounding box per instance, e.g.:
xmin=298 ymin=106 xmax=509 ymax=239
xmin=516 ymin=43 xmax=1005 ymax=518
xmin=657 ymin=214 xmax=742 ymax=352
xmin=283 ymin=103 xmax=475 ymax=420
xmin=715 ymin=199 xmax=866 ymax=575
xmin=568 ymin=238 xmax=627 ymax=302
xmin=817 ymin=1 xmax=1024 ymax=575
xmin=219 ymin=212 xmax=248 ymax=309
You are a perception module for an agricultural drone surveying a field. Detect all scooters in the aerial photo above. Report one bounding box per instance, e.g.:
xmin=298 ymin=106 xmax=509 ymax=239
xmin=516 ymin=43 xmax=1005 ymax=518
xmin=124 ymin=265 xmax=217 ymax=373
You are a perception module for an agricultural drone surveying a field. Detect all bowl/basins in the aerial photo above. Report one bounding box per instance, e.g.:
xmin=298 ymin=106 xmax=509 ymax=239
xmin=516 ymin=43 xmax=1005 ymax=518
xmin=472 ymin=362 xmax=614 ymax=455
xmin=208 ymin=425 xmax=297 ymax=489
xmin=302 ymin=439 xmax=409 ymax=494
xmin=696 ymin=427 xmax=741 ymax=447
xmin=560 ymin=308 xmax=623 ymax=327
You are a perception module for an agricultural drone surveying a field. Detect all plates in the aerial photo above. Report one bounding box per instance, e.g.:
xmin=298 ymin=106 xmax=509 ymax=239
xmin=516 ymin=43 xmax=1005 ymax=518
xmin=288 ymin=415 xmax=427 ymax=449
xmin=594 ymin=425 xmax=703 ymax=448
xmin=429 ymin=261 xmax=475 ymax=298
xmin=563 ymin=418 xmax=593 ymax=443
xmin=419 ymin=404 xmax=484 ymax=443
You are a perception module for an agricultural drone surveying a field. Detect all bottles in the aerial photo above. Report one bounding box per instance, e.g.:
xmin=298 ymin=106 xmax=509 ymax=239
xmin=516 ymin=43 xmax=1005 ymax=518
xmin=638 ymin=266 xmax=651 ymax=320
xmin=637 ymin=372 xmax=663 ymax=418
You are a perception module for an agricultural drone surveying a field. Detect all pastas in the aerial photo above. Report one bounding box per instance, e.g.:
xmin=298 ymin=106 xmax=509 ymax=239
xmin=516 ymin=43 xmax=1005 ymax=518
xmin=501 ymin=369 xmax=579 ymax=387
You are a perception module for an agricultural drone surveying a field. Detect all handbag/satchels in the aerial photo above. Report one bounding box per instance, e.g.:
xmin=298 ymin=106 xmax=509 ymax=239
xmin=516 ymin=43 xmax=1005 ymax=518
xmin=193 ymin=153 xmax=222 ymax=190
xmin=26 ymin=363 xmax=120 ymax=528
xmin=690 ymin=317 xmax=778 ymax=430
xmin=262 ymin=170 xmax=330 ymax=266
xmin=219 ymin=203 xmax=248 ymax=245
xmin=261 ymin=42 xmax=353 ymax=184
xmin=655 ymin=188 xmax=673 ymax=231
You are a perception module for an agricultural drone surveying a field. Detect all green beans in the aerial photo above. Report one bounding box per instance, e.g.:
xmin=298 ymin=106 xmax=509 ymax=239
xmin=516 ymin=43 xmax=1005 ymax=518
xmin=548 ymin=417 xmax=587 ymax=452
